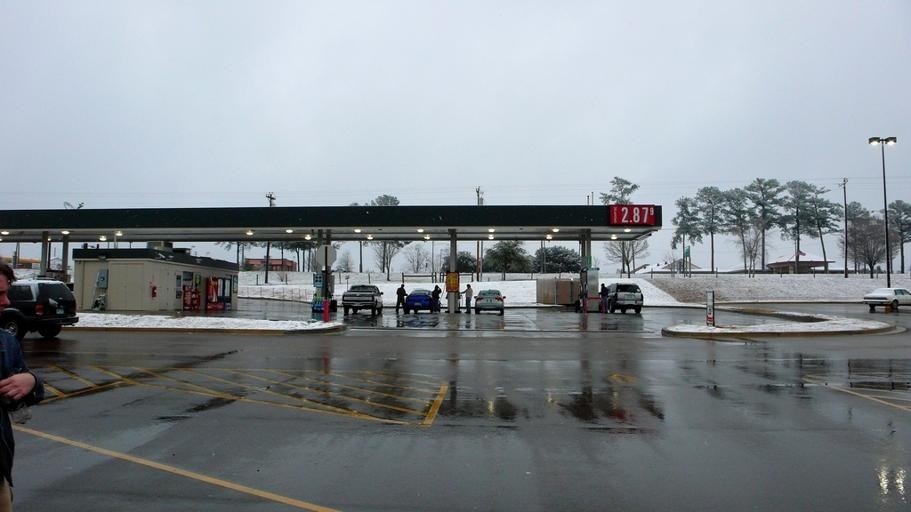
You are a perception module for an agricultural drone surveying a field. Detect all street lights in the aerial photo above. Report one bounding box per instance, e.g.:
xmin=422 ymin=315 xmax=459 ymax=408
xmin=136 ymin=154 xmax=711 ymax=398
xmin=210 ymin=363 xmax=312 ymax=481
xmin=868 ymin=136 xmax=897 ymax=288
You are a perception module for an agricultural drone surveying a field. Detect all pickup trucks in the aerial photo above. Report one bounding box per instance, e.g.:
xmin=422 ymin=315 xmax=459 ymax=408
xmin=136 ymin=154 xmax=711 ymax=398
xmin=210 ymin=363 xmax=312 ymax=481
xmin=342 ymin=285 xmax=384 ymax=316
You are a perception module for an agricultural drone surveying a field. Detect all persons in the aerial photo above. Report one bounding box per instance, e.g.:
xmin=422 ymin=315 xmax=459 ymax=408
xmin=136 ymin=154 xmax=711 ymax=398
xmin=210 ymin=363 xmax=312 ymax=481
xmin=0 ymin=260 xmax=44 ymax=512
xmin=599 ymin=283 xmax=610 ymax=313
xmin=395 ymin=284 xmax=407 ymax=314
xmin=430 ymin=285 xmax=441 ymax=312
xmin=460 ymin=284 xmax=472 ymax=314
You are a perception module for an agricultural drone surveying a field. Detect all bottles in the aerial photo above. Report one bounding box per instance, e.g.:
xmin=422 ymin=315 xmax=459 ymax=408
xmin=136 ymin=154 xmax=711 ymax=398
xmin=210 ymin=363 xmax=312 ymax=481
xmin=3 ymin=395 xmax=34 ymax=426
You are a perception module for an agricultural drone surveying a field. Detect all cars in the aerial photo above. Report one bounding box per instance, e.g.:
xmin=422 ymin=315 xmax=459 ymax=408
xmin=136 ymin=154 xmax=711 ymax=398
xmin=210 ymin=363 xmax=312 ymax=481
xmin=0 ymin=276 xmax=79 ymax=342
xmin=474 ymin=289 xmax=506 ymax=316
xmin=404 ymin=290 xmax=442 ymax=314
xmin=864 ymin=287 xmax=911 ymax=312
xmin=605 ymin=283 xmax=643 ymax=314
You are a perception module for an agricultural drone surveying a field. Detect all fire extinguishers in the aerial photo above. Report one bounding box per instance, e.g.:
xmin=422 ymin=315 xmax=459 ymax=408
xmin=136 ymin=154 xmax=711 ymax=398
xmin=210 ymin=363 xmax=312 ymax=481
xmin=152 ymin=285 xmax=157 ymax=297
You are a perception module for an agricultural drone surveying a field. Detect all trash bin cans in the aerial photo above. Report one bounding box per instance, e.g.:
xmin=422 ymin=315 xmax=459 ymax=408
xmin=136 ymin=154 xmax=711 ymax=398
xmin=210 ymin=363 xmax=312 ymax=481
xmin=331 ymin=300 xmax=337 ymax=312
